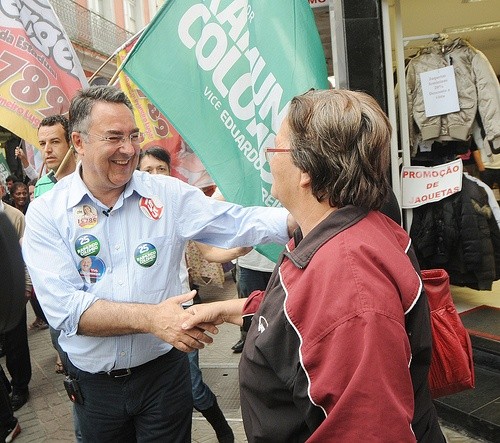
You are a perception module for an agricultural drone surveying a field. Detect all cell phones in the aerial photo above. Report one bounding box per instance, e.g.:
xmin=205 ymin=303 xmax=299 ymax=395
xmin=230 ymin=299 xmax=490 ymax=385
xmin=64 ymin=379 xmax=83 ymax=406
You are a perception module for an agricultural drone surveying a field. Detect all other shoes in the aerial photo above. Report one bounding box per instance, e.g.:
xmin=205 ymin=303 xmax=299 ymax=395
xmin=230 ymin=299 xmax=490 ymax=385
xmin=0 ymin=417 xmax=21 ymax=443
xmin=29 ymin=317 xmax=49 ymax=330
xmin=231 ymin=332 xmax=248 ymax=351
xmin=191 ymin=288 xmax=201 ymax=304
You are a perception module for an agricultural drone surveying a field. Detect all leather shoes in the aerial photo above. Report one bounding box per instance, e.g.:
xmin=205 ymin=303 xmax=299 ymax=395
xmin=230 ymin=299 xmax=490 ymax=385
xmin=10 ymin=387 xmax=30 ymax=411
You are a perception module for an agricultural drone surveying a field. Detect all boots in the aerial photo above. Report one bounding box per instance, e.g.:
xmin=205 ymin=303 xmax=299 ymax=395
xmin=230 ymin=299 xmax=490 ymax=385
xmin=197 ymin=394 xmax=234 ymax=443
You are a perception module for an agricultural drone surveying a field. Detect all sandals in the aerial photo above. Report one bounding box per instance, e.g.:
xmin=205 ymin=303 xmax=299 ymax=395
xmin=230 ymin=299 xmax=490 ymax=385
xmin=56 ymin=362 xmax=65 ymax=374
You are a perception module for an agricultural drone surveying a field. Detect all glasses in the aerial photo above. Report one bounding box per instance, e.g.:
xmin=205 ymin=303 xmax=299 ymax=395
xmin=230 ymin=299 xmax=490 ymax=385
xmin=78 ymin=130 xmax=145 ymax=147
xmin=261 ymin=147 xmax=296 ymax=160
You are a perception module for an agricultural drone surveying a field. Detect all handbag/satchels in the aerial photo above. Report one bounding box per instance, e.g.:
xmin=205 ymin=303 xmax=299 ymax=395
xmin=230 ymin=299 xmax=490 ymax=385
xmin=419 ymin=268 xmax=474 ymax=398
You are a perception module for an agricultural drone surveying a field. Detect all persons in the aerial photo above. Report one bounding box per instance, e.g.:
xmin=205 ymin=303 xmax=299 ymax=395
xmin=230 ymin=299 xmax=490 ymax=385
xmin=8 ymin=182 xmax=49 ymax=330
xmin=35 ymin=117 xmax=78 ymax=196
xmin=0 ymin=180 xmax=33 ymax=412
xmin=2 ymin=176 xmax=18 ymax=203
xmin=138 ymin=147 xmax=254 ymax=443
xmin=186 ymin=88 xmax=448 ymax=443
xmin=15 ymin=147 xmax=43 ymax=179
xmin=0 ymin=212 xmax=29 ymax=443
xmin=231 ymin=245 xmax=277 ymax=351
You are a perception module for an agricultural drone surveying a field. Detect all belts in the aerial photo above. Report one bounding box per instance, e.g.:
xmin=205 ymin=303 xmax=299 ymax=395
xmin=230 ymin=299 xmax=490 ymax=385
xmin=78 ymin=347 xmax=176 ymax=384
